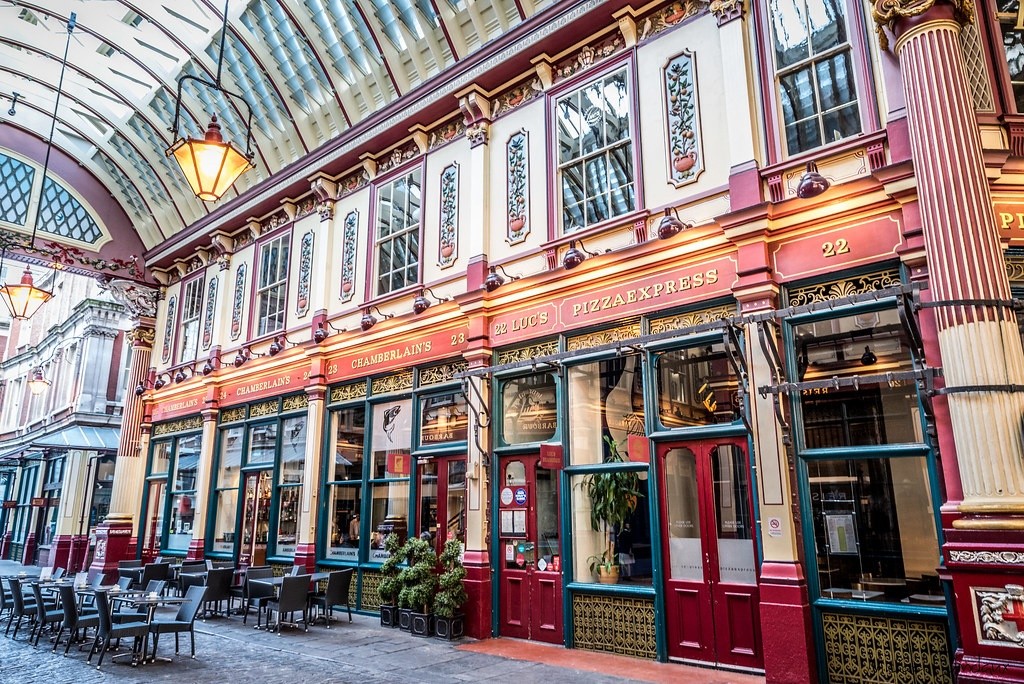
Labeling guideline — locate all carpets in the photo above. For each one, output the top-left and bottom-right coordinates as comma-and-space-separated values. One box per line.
450, 637, 766, 684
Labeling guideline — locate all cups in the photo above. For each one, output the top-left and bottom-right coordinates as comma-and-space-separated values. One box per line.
218, 567, 224, 569
44, 576, 50, 579
78, 582, 86, 586
285, 573, 290, 577
19, 572, 25, 574
55, 579, 62, 582
113, 584, 120, 589
149, 592, 158, 596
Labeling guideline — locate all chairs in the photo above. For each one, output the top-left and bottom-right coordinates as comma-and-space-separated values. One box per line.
817, 557, 946, 605
0, 557, 353, 670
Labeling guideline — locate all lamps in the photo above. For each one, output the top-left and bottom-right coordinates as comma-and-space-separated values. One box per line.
861, 498, 870, 508
165, 0, 258, 202
28, 337, 84, 395
135, 159, 833, 396
96, 483, 103, 490
704, 390, 717, 407
695, 380, 709, 394
860, 346, 878, 366
0, 12, 87, 322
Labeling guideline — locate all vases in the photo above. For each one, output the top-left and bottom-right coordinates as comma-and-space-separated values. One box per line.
222, 533, 234, 543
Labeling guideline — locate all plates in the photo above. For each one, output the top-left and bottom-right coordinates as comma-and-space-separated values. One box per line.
78, 586, 88, 588
147, 596, 160, 599
110, 589, 122, 592
55, 582, 64, 584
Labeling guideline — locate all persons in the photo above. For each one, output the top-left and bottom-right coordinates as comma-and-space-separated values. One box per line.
371, 532, 382, 550
333, 513, 361, 547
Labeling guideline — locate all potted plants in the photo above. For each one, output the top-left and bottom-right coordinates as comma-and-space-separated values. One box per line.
377, 531, 468, 642
572, 436, 644, 583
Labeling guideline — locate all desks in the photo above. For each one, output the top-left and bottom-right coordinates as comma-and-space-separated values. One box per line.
77, 589, 151, 652
859, 577, 922, 601
164, 563, 184, 605
26, 582, 76, 636
296, 572, 339, 626
248, 576, 299, 632
222, 568, 248, 616
19, 579, 70, 632
179, 571, 225, 622
110, 596, 193, 665
118, 567, 157, 609
49, 584, 121, 645
1, 575, 40, 623
824, 587, 886, 602
